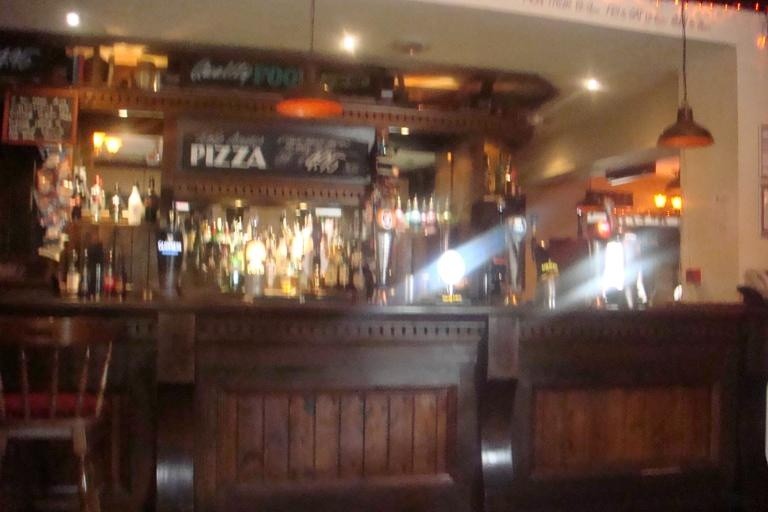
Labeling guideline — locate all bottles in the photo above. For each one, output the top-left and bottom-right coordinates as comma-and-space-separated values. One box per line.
59, 173, 456, 305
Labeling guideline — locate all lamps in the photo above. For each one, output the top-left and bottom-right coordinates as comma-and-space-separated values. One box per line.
276, 0, 344, 119
657, 6, 715, 150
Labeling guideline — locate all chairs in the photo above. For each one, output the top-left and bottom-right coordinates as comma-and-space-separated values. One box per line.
1, 314, 123, 511
